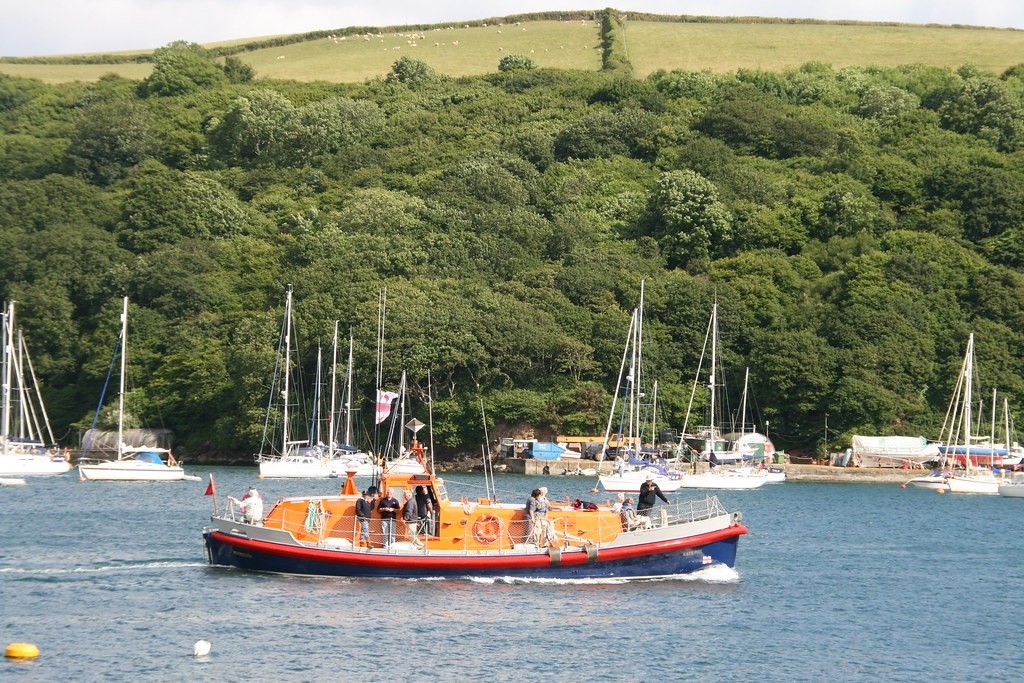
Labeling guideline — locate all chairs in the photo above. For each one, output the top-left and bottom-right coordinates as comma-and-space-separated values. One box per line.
621, 514, 637, 532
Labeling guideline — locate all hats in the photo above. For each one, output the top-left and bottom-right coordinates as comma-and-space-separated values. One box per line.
645, 475, 654, 480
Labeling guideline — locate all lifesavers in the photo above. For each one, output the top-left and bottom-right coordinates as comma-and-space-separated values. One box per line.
346, 461, 360, 470
764, 442, 772, 452
472, 512, 503, 544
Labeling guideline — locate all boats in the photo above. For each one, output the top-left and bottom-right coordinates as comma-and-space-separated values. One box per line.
203, 420, 750, 589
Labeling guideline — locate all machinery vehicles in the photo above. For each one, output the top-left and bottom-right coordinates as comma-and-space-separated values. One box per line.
557, 432, 741, 469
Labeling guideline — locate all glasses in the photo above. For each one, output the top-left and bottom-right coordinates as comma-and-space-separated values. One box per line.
363, 494, 368, 495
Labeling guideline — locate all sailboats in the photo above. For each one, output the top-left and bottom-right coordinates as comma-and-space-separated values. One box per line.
0, 298, 87, 484
78, 297, 188, 481
255, 283, 421, 478
598, 282, 793, 494
908, 331, 1024, 500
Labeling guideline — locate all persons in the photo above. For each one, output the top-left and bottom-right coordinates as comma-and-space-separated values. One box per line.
415, 485, 435, 535
524, 487, 563, 548
228, 487, 263, 525
377, 487, 400, 549
637, 474, 672, 516
356, 490, 377, 548
401, 490, 425, 550
620, 497, 651, 532
612, 493, 625, 513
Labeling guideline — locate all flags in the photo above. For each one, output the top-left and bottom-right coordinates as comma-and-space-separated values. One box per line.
376, 389, 398, 424
205, 479, 215, 495
